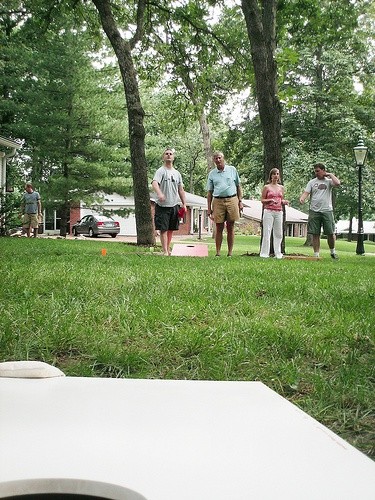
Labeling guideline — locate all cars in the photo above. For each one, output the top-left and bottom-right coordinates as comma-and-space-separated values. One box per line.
71, 214, 121, 239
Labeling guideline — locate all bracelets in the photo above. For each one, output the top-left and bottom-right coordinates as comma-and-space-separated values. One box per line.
38, 212, 42, 213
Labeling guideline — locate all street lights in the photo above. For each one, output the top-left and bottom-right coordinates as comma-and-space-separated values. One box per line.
352, 138, 369, 255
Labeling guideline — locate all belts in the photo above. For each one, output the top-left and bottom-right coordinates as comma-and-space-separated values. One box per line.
215, 195, 236, 198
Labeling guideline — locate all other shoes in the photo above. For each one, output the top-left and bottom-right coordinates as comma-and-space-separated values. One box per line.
330, 253, 338, 259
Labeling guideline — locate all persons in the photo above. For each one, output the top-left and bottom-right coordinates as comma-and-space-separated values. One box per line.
260, 168, 289, 259
151, 149, 187, 256
19, 183, 42, 238
299, 163, 341, 259
206, 151, 244, 257
